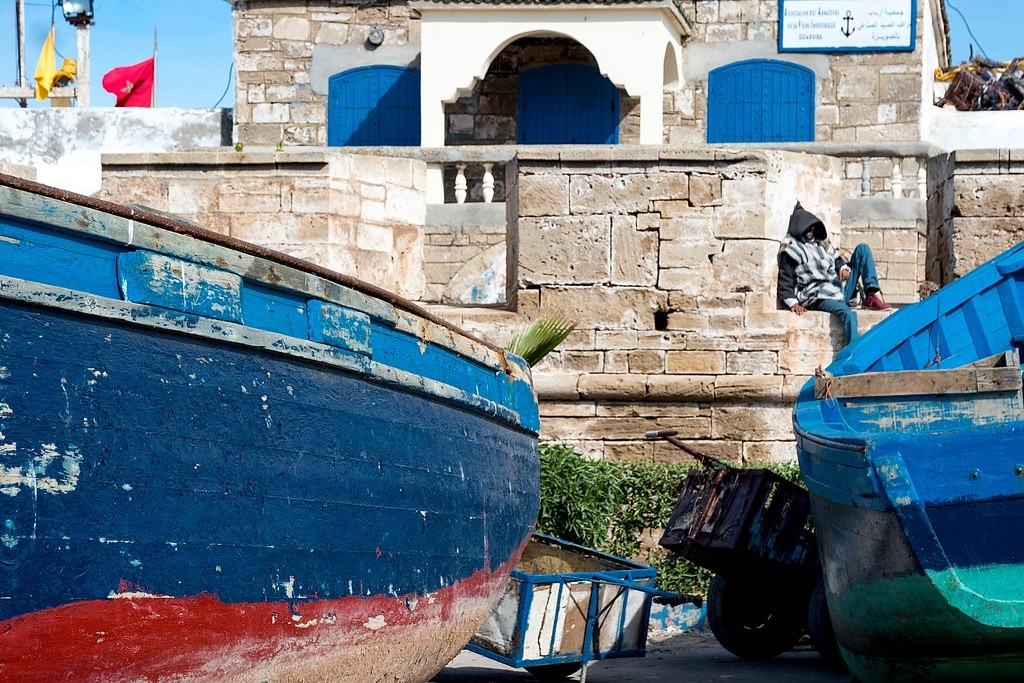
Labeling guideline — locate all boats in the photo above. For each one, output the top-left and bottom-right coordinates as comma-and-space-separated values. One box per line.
793, 242, 1023, 683
0, 173, 542, 683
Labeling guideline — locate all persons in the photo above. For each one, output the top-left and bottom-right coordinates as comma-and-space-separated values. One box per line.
778, 204, 890, 350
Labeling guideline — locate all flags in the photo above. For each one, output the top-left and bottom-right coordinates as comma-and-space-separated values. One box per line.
103, 58, 154, 108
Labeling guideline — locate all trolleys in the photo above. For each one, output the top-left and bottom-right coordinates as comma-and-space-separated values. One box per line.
645, 426, 849, 672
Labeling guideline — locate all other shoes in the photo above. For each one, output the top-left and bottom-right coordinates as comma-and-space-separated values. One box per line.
861, 292, 893, 311
